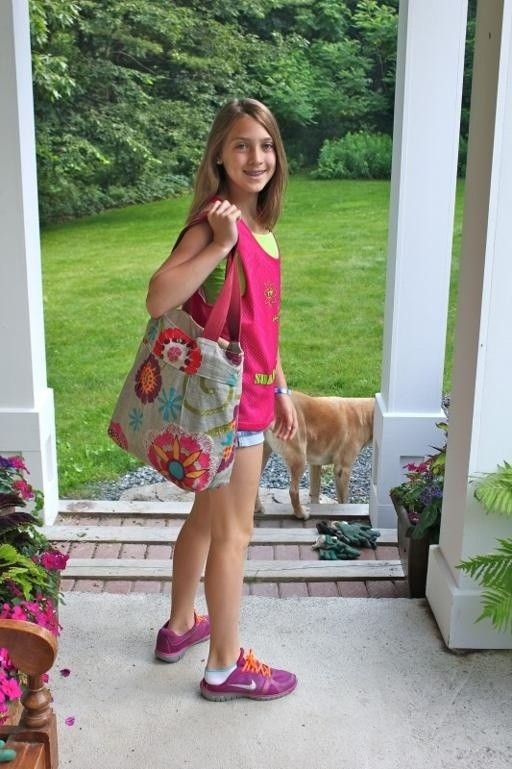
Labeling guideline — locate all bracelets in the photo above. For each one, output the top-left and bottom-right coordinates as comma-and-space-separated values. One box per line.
275, 387, 293, 396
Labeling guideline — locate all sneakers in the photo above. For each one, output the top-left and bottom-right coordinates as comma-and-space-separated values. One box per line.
201, 647, 297, 701
154, 609, 210, 662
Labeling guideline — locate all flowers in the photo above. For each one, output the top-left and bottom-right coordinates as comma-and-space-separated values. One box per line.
0, 456, 68, 725
390, 397, 448, 541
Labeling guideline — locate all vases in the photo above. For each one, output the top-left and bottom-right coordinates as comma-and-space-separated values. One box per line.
389, 489, 440, 597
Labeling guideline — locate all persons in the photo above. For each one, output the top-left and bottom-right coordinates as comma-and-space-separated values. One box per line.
144, 93, 297, 701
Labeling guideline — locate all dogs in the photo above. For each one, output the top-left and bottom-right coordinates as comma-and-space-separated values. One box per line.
257, 388, 376, 522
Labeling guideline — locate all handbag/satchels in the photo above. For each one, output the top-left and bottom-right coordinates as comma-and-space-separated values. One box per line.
108, 212, 244, 493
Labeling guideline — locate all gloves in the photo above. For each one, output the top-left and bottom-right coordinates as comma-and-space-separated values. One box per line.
312, 519, 381, 560
